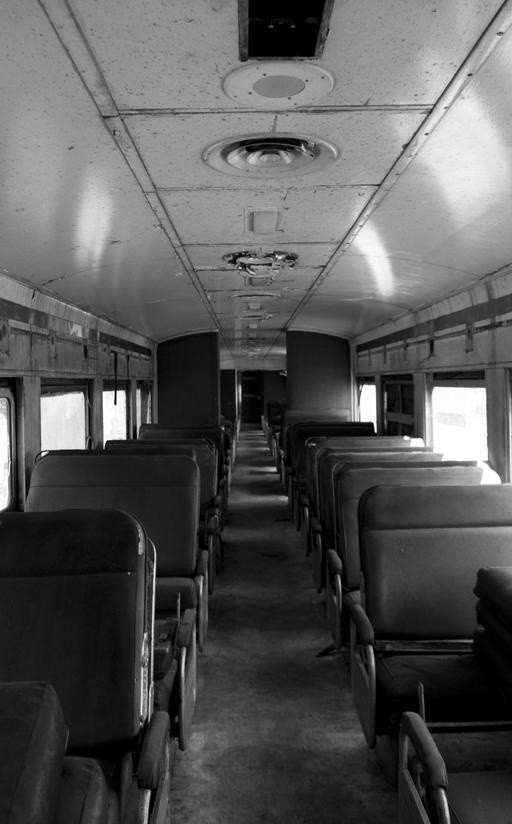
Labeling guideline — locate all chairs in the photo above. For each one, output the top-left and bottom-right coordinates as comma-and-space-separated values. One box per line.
0, 414, 236, 824
261, 400, 512, 824
